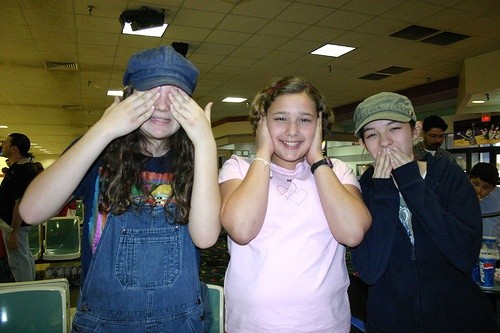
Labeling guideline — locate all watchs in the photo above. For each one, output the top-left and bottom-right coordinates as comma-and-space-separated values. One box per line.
310, 156, 334, 174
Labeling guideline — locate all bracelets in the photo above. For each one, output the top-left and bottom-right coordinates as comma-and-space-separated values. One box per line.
249, 157, 272, 166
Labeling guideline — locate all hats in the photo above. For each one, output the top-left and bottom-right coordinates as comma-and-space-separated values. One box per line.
353, 91, 416, 138
122, 46, 200, 98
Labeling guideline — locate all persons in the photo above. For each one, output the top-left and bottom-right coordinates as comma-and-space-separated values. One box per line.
0, 132, 42, 283
414, 116, 459, 164
18, 45, 221, 333
216, 75, 375, 333
467, 162, 500, 237
347, 92, 500, 333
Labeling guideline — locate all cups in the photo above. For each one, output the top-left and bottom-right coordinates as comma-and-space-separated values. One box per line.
479, 255, 496, 289
483, 236, 496, 255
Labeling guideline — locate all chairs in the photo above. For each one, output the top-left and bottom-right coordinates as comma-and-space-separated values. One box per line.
43, 216, 82, 262
76, 200, 85, 224
27, 224, 42, 260
0, 278, 70, 333
202, 284, 225, 333
